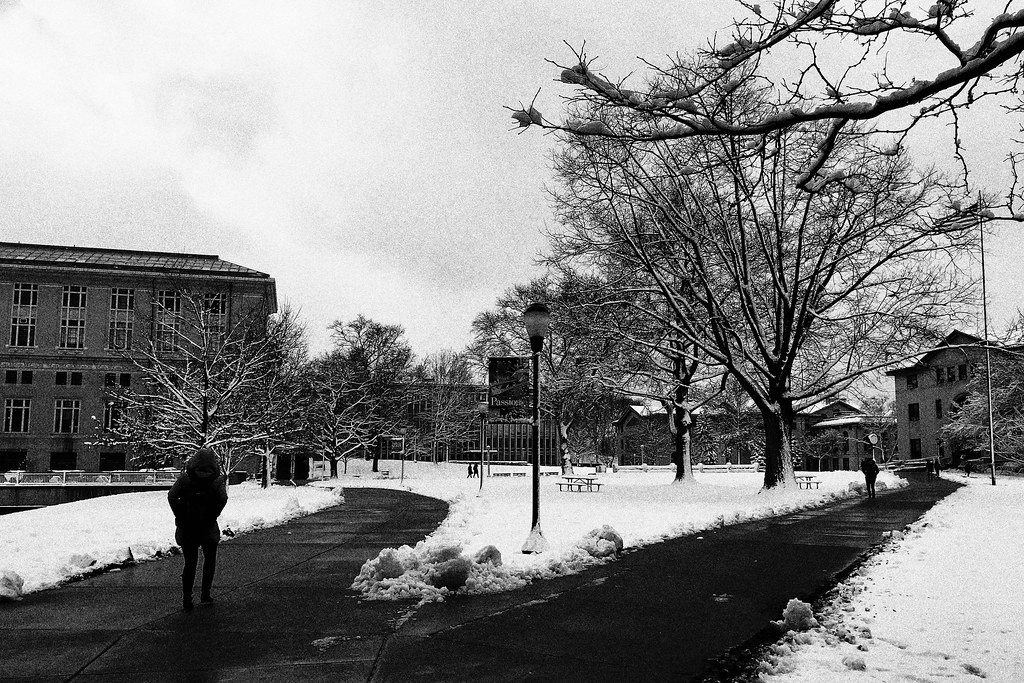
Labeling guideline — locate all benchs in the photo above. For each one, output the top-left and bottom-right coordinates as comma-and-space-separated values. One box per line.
797, 481, 822, 484
576, 481, 604, 486
555, 482, 586, 487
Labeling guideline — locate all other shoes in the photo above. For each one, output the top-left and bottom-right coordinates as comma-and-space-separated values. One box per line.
183, 602, 194, 610
200, 594, 214, 603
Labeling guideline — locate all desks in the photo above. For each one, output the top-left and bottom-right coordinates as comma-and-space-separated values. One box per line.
559, 476, 600, 492
795, 476, 820, 489
9, 470, 27, 485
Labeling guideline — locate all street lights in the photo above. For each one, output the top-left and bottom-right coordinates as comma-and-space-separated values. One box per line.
477, 399, 490, 489
521, 302, 552, 555
399, 423, 408, 484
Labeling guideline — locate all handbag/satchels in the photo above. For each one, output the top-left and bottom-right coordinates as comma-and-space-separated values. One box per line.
175, 526, 181, 546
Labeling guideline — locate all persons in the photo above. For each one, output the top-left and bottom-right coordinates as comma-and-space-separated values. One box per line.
168, 449, 228, 612
467, 462, 479, 478
965, 462, 971, 477
926, 458, 940, 481
861, 454, 879, 499
